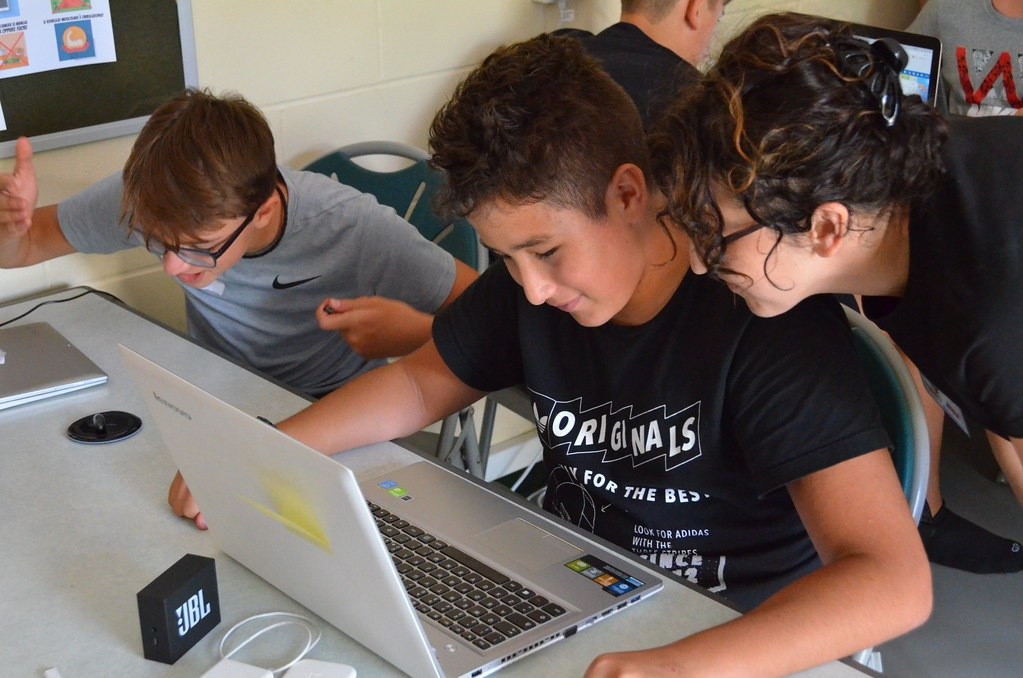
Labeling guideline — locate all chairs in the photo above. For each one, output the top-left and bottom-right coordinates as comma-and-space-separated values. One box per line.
831, 295, 932, 671
295, 140, 489, 487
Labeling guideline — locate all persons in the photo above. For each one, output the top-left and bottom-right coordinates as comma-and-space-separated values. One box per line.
167, 0, 1023, 678
0, 87, 481, 401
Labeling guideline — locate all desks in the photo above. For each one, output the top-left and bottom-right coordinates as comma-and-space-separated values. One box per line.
0, 282, 894, 678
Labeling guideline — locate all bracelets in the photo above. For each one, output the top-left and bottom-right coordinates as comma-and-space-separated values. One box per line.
256, 415, 279, 432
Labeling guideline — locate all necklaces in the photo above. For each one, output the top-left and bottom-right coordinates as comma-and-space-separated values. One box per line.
241, 182, 289, 259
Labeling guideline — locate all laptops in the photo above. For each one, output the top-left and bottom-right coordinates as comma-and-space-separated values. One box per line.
0, 322, 109, 410
786, 11, 942, 108
118, 343, 663, 677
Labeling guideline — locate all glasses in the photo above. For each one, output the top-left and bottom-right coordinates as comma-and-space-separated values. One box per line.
128, 205, 260, 269
669, 200, 804, 267
